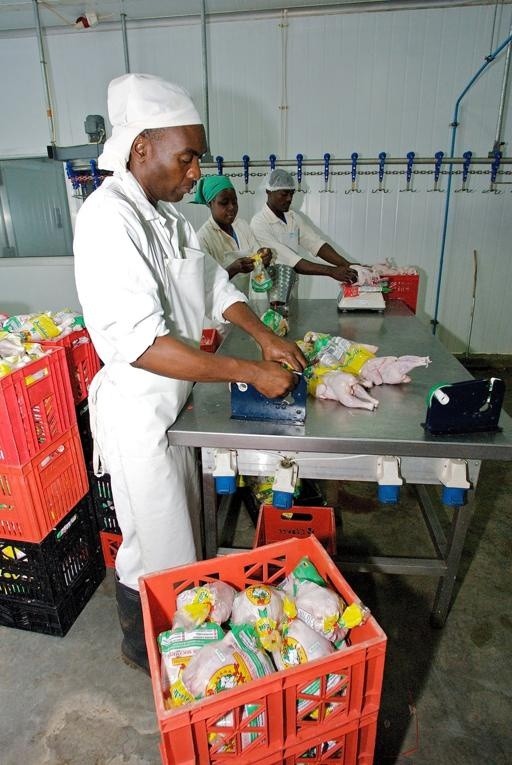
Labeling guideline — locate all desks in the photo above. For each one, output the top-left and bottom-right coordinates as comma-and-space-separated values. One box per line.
163, 298, 512, 632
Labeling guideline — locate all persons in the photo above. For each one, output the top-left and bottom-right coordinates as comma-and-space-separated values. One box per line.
191, 172, 276, 352
71, 73, 313, 682
248, 168, 371, 303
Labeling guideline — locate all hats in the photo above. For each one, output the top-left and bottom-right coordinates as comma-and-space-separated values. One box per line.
105, 72, 203, 128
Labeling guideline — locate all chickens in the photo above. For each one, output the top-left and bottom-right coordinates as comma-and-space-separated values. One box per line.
313, 369, 383, 412
359, 351, 433, 388
346, 253, 418, 289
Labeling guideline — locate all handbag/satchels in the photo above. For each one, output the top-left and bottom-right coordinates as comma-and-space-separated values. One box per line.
422, 376, 505, 435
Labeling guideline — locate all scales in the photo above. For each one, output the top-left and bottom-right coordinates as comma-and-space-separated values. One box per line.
336, 291, 386, 313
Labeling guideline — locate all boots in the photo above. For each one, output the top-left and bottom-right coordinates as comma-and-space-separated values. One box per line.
113, 568, 151, 676
